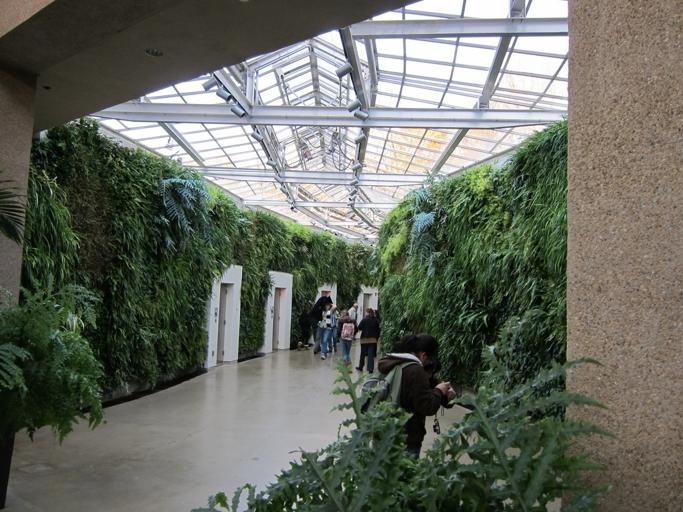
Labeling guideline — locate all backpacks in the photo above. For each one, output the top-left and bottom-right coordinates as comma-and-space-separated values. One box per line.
360, 361, 417, 421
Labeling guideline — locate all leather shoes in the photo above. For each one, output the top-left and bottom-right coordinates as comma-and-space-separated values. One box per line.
356, 366, 362, 370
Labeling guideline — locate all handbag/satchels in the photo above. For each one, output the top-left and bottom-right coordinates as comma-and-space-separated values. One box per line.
341, 322, 355, 341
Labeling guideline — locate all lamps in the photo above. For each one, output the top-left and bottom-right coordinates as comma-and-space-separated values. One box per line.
201, 63, 368, 220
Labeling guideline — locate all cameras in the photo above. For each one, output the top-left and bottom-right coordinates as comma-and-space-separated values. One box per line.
431, 421, 441, 434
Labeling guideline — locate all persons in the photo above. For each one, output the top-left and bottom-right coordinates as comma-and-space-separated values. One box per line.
336, 310, 357, 368
346, 303, 359, 341
372, 309, 382, 358
421, 356, 457, 403
375, 331, 451, 482
297, 289, 339, 360
356, 308, 380, 373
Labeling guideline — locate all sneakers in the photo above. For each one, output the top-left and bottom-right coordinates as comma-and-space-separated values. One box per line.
343, 359, 351, 366
321, 347, 337, 360
